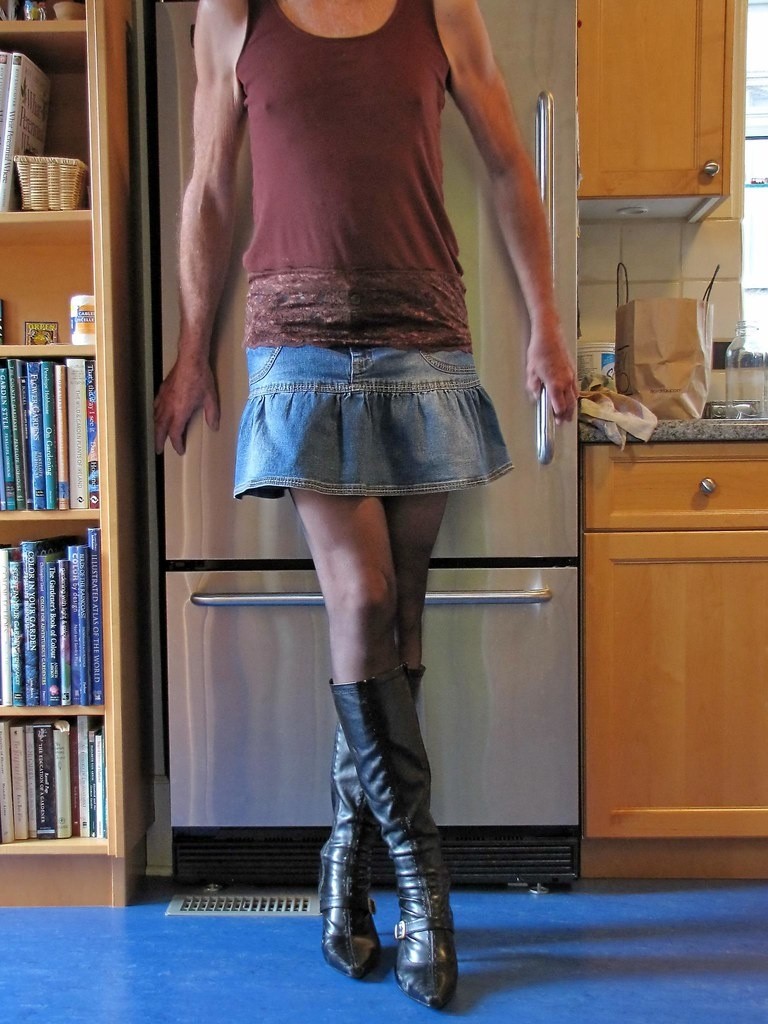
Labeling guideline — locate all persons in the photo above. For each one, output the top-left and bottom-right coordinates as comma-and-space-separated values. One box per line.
153, 0, 579, 1008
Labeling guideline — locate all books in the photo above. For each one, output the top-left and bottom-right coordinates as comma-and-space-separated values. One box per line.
0, 356, 112, 843
0, 52, 52, 210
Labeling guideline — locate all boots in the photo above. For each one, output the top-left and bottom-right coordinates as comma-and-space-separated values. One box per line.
328, 661, 458, 1010
317, 663, 428, 978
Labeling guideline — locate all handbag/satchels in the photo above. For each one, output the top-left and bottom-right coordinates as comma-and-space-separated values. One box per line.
608, 259, 720, 418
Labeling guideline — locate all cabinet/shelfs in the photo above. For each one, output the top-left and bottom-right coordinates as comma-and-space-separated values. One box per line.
576, 442, 767, 877
0, 0, 167, 907
576, 0, 736, 225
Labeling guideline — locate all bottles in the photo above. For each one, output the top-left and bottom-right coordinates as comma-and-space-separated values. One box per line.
725, 322, 768, 418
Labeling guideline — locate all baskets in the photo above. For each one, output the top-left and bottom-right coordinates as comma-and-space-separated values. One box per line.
12, 154, 89, 211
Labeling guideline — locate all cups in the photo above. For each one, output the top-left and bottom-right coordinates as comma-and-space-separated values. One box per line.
580, 342, 614, 380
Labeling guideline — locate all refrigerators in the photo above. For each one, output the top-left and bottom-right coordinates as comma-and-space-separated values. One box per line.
156, 0, 579, 894
